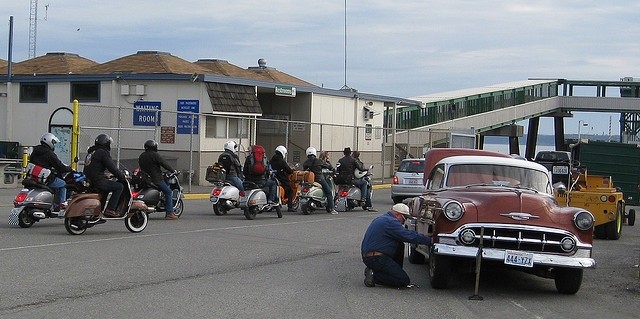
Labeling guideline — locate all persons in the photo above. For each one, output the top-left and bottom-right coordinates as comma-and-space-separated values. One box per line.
336, 148, 369, 209
84, 133, 125, 217
269, 145, 296, 212
319, 150, 337, 173
21, 131, 71, 211
303, 146, 339, 214
139, 140, 179, 220
218, 140, 244, 192
351, 150, 366, 169
243, 146, 277, 206
361, 203, 431, 290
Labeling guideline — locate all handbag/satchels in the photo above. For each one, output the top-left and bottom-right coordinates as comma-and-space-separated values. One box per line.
25, 156, 53, 183
293, 158, 316, 184
206, 162, 226, 181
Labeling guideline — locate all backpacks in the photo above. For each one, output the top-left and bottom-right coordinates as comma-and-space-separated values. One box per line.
248, 144, 267, 175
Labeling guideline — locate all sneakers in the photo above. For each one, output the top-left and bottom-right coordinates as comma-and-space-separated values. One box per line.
105, 208, 121, 217
364, 268, 375, 287
165, 214, 177, 220
267, 200, 279, 206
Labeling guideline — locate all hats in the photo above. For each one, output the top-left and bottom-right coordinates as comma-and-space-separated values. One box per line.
390, 203, 410, 216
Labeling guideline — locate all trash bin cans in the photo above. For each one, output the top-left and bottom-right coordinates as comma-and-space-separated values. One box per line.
182, 169, 195, 185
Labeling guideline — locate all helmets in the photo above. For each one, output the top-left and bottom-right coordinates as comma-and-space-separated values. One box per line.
95, 134, 113, 146
275, 146, 287, 159
306, 147, 317, 159
144, 140, 159, 151
40, 133, 61, 150
224, 140, 238, 153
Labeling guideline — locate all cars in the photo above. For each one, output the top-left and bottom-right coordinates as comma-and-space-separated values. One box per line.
402, 147, 595, 294
391, 159, 431, 204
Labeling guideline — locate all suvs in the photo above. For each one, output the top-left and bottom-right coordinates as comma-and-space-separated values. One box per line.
531, 151, 570, 185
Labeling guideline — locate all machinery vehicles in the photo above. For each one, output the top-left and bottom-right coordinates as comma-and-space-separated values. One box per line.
552, 159, 635, 239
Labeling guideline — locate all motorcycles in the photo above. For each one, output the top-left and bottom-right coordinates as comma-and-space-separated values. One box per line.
210, 180, 245, 216
239, 161, 283, 220
334, 165, 375, 211
298, 163, 341, 215
130, 170, 184, 218
62, 169, 149, 235
8, 157, 86, 228
280, 163, 300, 212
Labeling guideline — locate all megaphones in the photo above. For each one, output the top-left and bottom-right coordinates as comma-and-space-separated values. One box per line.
367, 100, 374, 108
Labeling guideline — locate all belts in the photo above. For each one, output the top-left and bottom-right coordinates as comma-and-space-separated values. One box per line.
364, 251, 384, 257
